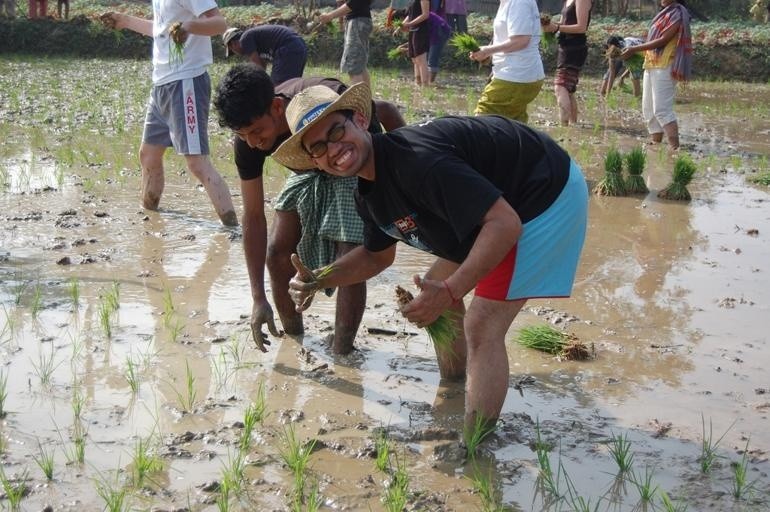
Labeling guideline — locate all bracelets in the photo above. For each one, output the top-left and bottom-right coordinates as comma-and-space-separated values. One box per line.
442, 277, 459, 305
553, 25, 559, 33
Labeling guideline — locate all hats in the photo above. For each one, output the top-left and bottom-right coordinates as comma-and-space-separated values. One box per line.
270, 81, 372, 170
221, 27, 240, 58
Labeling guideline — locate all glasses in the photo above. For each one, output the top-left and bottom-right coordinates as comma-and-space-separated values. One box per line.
304, 112, 353, 158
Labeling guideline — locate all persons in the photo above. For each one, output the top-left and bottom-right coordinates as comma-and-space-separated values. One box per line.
27, 2, 50, 22
223, 24, 311, 88
385, 1, 468, 86
1, 0, 18, 21
319, 1, 372, 84
57, 1, 71, 21
213, 66, 411, 362
543, 1, 593, 125
268, 80, 590, 448
601, 36, 647, 96
469, 1, 547, 122
618, 2, 689, 150
85, 1, 241, 233
